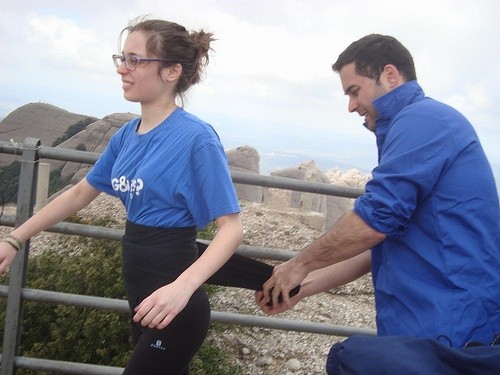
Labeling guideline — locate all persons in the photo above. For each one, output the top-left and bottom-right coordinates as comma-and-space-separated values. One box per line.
0, 14, 244, 375
255, 32, 500, 375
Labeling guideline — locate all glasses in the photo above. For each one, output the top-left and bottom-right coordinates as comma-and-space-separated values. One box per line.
113, 55, 176, 71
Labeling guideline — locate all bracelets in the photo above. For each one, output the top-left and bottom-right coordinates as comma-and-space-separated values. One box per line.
3, 235, 23, 251
